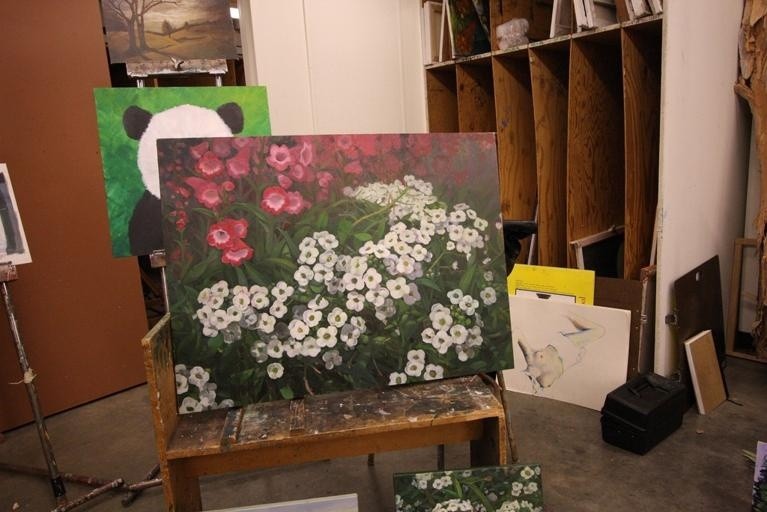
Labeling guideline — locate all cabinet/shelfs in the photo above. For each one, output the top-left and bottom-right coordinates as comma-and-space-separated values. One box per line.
420, 0, 752, 379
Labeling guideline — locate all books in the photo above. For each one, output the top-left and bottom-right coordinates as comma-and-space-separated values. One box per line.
684, 329, 727, 415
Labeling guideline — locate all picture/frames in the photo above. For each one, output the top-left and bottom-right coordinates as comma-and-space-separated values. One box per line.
549, 0, 663, 38
568, 226, 625, 278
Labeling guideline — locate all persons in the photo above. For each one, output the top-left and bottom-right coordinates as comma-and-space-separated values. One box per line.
513, 310, 607, 391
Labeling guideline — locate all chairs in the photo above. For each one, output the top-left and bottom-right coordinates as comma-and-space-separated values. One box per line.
140, 249, 508, 511
